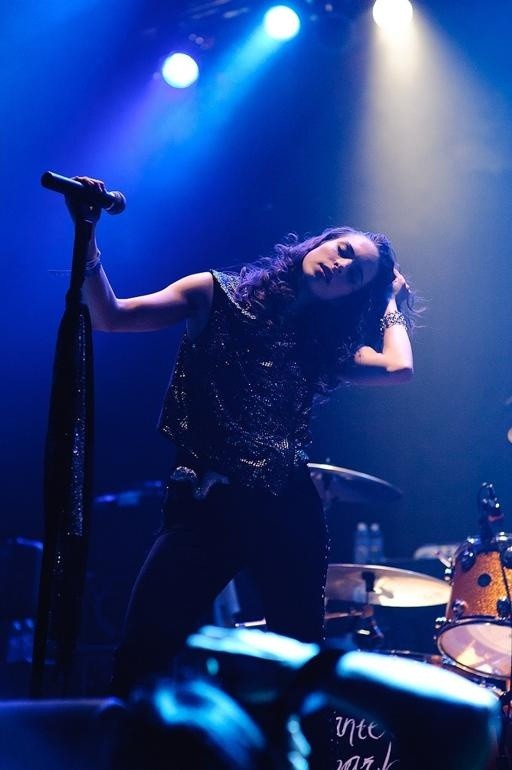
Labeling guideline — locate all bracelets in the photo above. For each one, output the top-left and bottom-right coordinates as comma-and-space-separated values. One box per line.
379, 310, 407, 330
81, 247, 104, 276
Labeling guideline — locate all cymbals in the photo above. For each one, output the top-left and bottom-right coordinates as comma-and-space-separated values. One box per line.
307, 462, 402, 513
326, 562, 453, 608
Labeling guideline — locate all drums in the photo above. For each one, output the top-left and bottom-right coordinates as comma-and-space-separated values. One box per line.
433, 531, 512, 681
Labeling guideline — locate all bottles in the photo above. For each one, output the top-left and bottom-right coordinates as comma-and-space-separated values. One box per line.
6, 618, 35, 664
353, 522, 370, 564
370, 523, 386, 564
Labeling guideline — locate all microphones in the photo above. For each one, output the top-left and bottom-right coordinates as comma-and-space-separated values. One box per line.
41, 171, 126, 215
488, 486, 504, 536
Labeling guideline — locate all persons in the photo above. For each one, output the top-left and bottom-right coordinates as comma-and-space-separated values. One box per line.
65, 174, 417, 697
